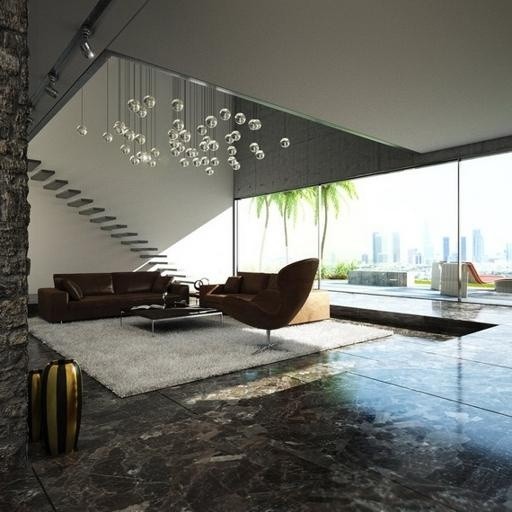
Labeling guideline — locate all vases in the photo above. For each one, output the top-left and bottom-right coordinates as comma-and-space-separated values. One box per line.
24, 368, 46, 444
38, 357, 84, 454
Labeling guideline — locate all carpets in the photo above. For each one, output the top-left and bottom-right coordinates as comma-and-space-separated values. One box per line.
26, 299, 398, 398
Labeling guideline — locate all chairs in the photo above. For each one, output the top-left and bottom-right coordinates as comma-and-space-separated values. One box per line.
218, 256, 319, 354
459, 257, 505, 294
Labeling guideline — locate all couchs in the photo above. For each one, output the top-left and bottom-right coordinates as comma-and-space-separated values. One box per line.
37, 271, 181, 325
196, 271, 277, 309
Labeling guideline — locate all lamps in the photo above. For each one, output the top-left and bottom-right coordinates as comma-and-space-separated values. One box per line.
45, 70, 62, 98
73, 28, 96, 63
76, 55, 294, 178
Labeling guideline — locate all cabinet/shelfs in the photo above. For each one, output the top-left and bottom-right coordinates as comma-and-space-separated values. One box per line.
344, 270, 409, 288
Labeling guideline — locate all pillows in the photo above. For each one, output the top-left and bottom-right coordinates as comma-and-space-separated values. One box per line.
151, 273, 173, 292
52, 276, 84, 300
220, 275, 244, 295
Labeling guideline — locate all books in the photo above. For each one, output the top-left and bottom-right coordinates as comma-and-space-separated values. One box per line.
131, 303, 166, 311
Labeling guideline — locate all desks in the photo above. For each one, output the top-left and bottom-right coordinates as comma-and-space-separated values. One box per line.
287, 286, 331, 325
494, 277, 512, 297
